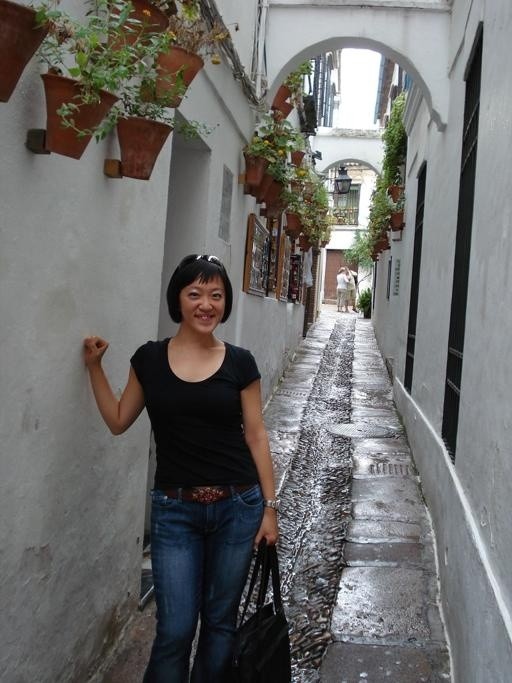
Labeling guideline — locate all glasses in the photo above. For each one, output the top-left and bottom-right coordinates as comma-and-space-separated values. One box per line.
184, 254, 220, 264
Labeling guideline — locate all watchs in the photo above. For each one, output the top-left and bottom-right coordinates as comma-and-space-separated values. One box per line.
262, 499, 282, 511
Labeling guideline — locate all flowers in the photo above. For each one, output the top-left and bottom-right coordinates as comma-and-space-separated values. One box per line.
242, 121, 338, 251
0, 0, 239, 140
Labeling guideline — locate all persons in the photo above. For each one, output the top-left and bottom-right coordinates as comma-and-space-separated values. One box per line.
336, 265, 358, 313
84, 254, 289, 683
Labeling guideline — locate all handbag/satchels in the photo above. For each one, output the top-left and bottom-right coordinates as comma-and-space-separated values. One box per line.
226, 537, 291, 683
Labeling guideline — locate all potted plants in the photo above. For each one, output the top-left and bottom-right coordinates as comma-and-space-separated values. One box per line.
342, 89, 409, 273
272, 59, 315, 121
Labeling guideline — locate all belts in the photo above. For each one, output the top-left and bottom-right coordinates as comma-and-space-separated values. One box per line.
162, 481, 249, 503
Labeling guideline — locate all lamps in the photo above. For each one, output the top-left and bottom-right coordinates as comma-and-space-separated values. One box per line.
334, 165, 353, 194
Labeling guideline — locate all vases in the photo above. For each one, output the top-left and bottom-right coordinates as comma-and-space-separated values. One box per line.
41, 73, 118, 162
118, 114, 175, 181
